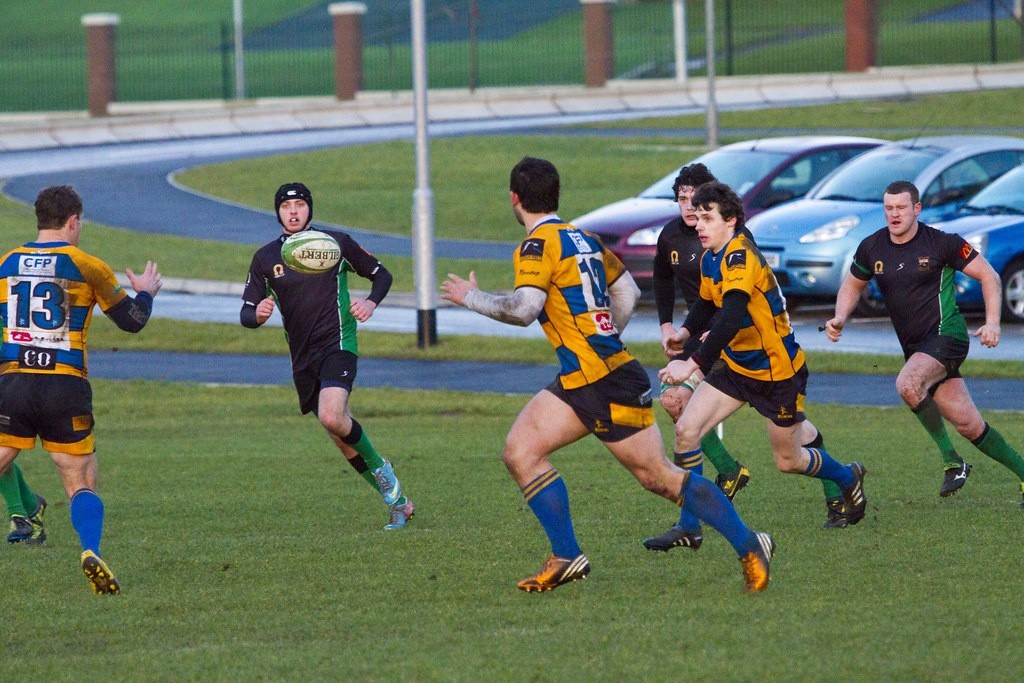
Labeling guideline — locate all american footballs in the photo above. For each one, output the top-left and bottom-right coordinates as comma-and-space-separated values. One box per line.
281, 230, 342, 272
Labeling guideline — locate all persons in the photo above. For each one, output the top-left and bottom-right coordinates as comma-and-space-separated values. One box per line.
439, 156, 776, 591
0, 183, 161, 594
643, 183, 866, 553
826, 181, 1023, 506
654, 162, 847, 529
241, 182, 417, 531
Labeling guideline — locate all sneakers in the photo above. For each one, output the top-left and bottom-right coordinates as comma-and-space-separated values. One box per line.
716, 462, 750, 501
515, 552, 592, 592
842, 461, 867, 525
7, 494, 48, 544
823, 496, 848, 528
739, 533, 777, 594
383, 497, 414, 531
643, 527, 705, 551
369, 458, 402, 505
938, 458, 972, 497
1017, 482, 1024, 509
80, 549, 121, 594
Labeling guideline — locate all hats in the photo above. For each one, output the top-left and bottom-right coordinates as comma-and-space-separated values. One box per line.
273, 182, 313, 233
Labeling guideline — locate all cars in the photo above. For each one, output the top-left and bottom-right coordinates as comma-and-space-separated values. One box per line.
744, 135, 1024, 317
569, 134, 895, 302
864, 162, 1024, 323
